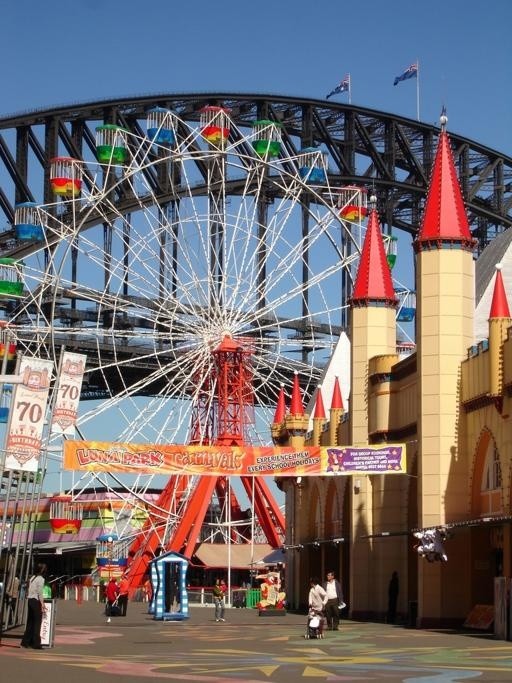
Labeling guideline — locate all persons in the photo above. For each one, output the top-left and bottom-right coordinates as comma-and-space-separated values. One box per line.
20, 562, 52, 650
105, 573, 130, 616
6, 574, 21, 625
307, 569, 344, 634
213, 577, 228, 622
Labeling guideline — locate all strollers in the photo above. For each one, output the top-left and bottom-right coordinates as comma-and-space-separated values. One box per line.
303, 611, 328, 641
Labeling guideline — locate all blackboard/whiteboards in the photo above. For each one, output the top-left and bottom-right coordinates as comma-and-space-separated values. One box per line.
39, 599, 55, 645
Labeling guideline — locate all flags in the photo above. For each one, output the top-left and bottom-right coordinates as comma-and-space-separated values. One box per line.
325, 73, 350, 101
393, 64, 417, 85
